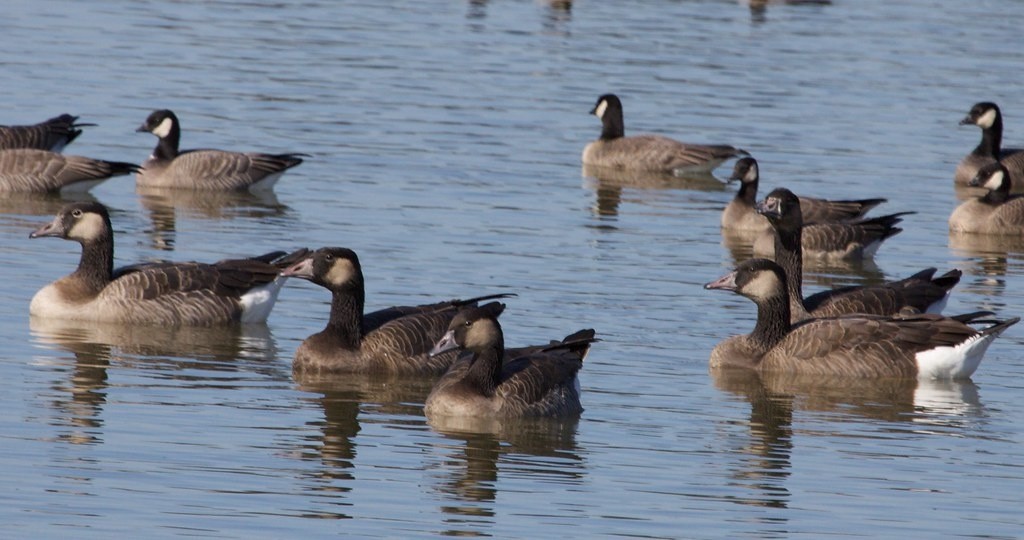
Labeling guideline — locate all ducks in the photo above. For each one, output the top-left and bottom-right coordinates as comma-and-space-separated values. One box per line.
0, 94, 1024, 418
